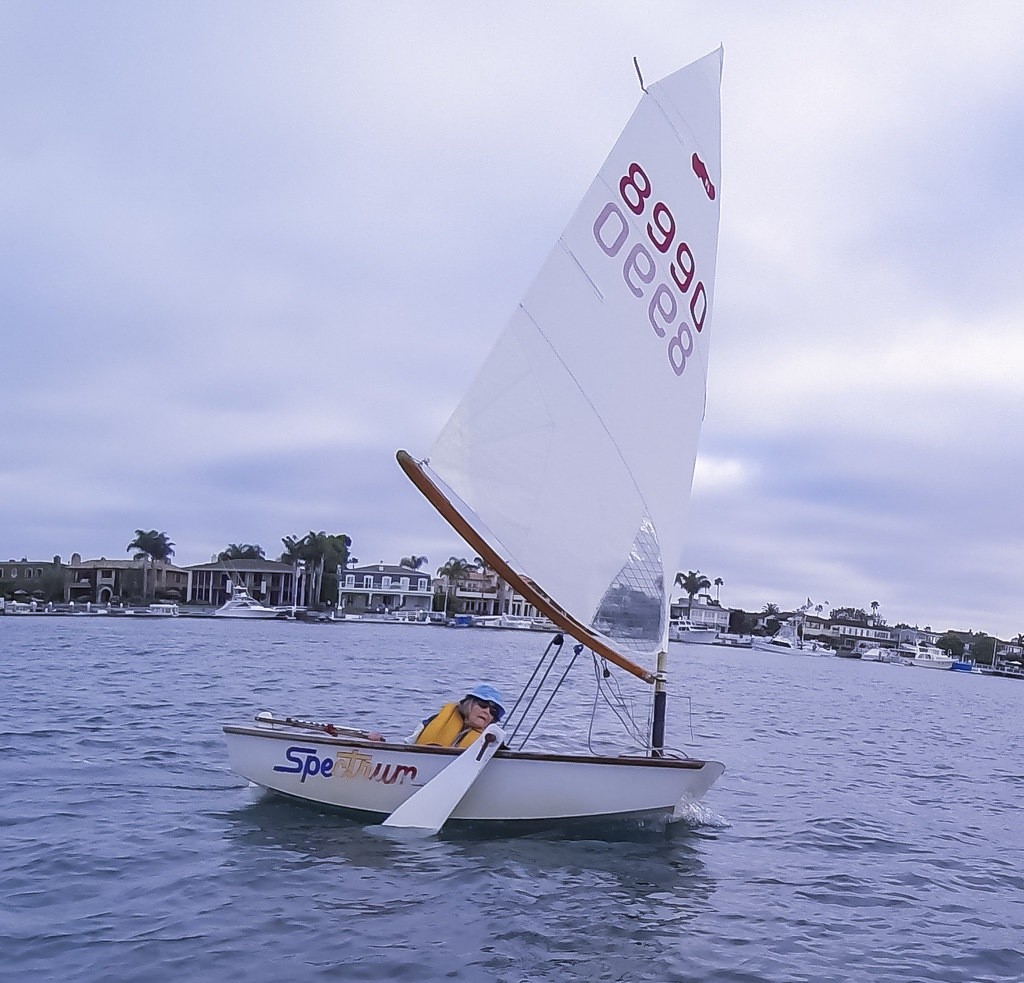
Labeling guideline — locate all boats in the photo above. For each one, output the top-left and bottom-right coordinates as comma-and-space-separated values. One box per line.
667, 617, 721, 644
723, 610, 984, 675
445, 611, 567, 636
213, 585, 288, 619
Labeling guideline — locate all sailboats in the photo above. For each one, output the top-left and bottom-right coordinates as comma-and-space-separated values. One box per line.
219, 30, 731, 836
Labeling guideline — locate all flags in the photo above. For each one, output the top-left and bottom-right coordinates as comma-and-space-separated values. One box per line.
297, 559, 306, 570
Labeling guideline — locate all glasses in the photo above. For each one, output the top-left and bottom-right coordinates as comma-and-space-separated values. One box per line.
477, 699, 499, 717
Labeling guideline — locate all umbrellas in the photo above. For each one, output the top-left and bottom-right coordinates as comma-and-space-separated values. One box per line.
166, 590, 179, 600
14, 589, 28, 595
32, 589, 45, 594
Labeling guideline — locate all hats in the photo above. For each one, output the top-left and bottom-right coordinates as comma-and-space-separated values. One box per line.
467, 682, 505, 718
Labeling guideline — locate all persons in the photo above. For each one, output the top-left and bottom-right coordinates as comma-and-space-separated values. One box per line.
327, 599, 331, 609
366, 684, 505, 748
813, 644, 817, 651
801, 642, 803, 649
